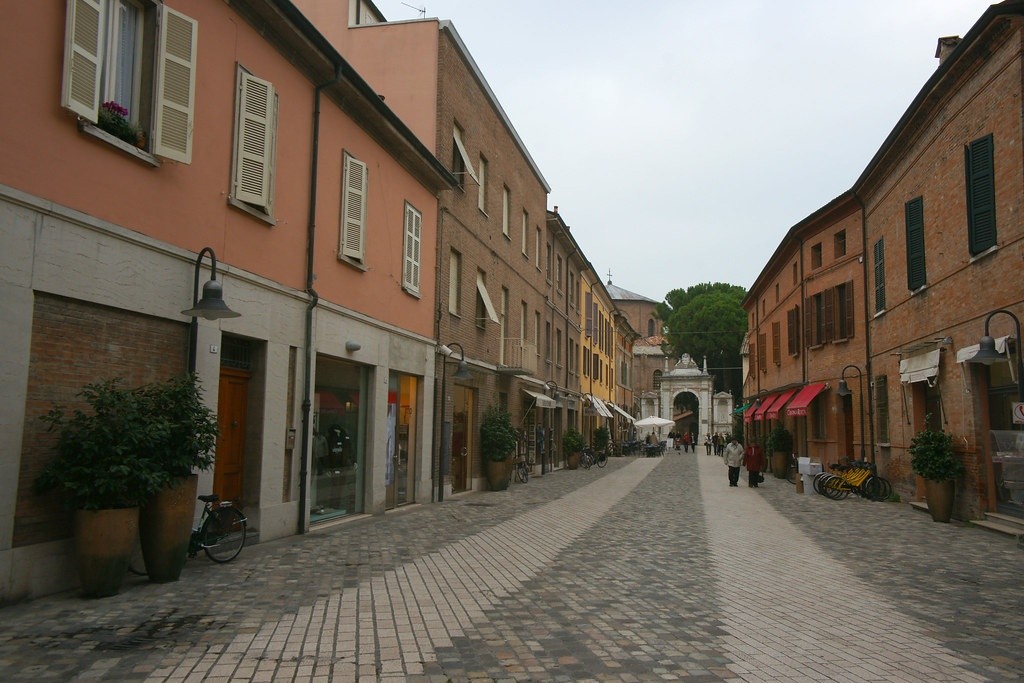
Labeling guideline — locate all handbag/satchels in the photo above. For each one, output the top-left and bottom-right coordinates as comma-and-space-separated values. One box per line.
758, 472, 764, 483
704, 442, 707, 445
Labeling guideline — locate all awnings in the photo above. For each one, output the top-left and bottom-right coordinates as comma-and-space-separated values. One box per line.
522, 388, 556, 409
786, 383, 825, 415
610, 402, 636, 420
453, 135, 481, 186
729, 386, 798, 421
476, 270, 501, 325
586, 395, 613, 418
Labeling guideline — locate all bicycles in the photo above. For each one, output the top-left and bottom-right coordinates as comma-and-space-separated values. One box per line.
512, 452, 531, 483
191, 493, 248, 563
579, 444, 608, 471
813, 455, 892, 502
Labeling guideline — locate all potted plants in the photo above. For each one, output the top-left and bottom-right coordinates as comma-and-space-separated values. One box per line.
561, 427, 585, 470
766, 425, 792, 479
907, 412, 965, 522
36, 365, 225, 599
478, 405, 523, 491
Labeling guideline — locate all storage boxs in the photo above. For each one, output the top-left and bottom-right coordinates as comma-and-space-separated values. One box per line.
797, 456, 822, 475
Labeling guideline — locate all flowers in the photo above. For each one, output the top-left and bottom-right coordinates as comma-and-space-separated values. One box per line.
97, 100, 144, 134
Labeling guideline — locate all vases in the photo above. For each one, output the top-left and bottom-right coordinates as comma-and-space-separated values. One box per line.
89, 113, 137, 146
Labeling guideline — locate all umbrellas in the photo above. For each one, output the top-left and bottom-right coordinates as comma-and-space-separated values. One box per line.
634, 416, 676, 434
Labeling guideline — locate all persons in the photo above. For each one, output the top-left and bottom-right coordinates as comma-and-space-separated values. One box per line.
650, 432, 657, 445
742, 439, 766, 489
311, 424, 351, 514
667, 429, 697, 455
722, 435, 744, 487
704, 432, 732, 457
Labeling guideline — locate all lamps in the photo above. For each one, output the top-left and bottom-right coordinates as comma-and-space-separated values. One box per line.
941, 335, 953, 345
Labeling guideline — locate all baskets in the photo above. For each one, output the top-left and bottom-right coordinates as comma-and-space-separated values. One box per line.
514, 456, 526, 464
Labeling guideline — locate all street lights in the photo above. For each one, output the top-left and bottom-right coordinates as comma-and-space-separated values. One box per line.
834, 365, 865, 468
542, 380, 563, 475
438, 343, 475, 502
756, 389, 772, 473
968, 310, 1023, 403
581, 392, 595, 446
181, 246, 243, 386
